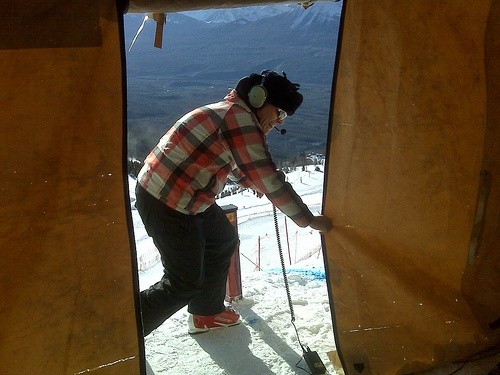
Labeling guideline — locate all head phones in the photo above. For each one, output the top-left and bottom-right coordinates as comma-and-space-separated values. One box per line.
248, 72, 269, 108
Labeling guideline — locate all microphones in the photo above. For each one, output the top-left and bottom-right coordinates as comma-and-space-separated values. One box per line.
274, 126, 287, 135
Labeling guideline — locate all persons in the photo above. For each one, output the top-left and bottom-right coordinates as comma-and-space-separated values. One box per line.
134, 70, 333, 337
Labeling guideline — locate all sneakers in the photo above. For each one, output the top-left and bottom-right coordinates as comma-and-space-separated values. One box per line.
191, 307, 240, 328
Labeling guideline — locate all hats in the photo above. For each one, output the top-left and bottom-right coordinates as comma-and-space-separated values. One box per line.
250, 69, 303, 115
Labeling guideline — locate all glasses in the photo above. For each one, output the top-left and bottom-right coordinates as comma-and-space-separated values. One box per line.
272, 103, 286, 121
